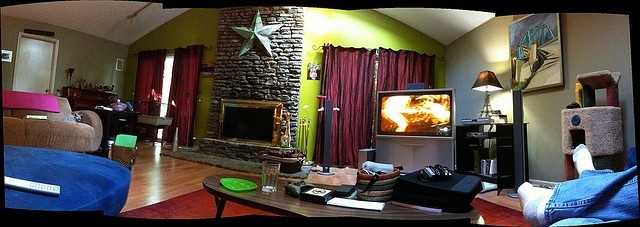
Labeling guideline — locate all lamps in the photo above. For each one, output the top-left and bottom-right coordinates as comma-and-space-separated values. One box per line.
69, 68, 74, 87
471, 71, 503, 118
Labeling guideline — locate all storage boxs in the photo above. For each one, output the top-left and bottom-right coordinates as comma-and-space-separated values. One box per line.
266, 155, 304, 173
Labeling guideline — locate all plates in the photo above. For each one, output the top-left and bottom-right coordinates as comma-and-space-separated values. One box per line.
220, 177, 257, 191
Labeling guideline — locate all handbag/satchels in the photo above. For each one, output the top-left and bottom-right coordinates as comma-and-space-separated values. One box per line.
392, 167, 483, 213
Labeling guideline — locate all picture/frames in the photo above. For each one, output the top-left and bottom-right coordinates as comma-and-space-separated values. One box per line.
508, 13, 564, 92
1, 50, 13, 63
116, 58, 124, 71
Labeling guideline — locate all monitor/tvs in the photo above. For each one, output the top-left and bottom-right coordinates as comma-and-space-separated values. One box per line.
374, 88, 456, 139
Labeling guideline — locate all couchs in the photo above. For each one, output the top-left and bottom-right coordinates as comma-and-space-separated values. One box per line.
4, 146, 130, 217
2, 89, 103, 153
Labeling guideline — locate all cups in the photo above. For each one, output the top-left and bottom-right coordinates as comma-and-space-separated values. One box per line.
262, 161, 281, 193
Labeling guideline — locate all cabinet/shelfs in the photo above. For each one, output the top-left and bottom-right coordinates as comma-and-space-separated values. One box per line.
64, 87, 119, 112
455, 123, 529, 197
376, 136, 454, 173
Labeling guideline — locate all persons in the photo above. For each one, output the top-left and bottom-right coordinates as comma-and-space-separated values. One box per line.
517, 143, 640, 225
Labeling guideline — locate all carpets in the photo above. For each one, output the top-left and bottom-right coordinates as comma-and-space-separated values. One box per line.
471, 198, 531, 226
119, 189, 534, 227
162, 150, 312, 178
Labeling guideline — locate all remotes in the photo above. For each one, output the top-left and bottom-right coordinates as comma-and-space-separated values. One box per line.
4, 175, 62, 198
336, 184, 354, 196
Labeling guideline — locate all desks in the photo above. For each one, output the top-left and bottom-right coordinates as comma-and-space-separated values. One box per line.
139, 115, 173, 147
81, 107, 139, 151
561, 105, 622, 182
202, 174, 482, 219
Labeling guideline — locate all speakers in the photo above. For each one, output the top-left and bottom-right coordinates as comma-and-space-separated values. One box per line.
507, 90, 526, 198
317, 99, 336, 175
